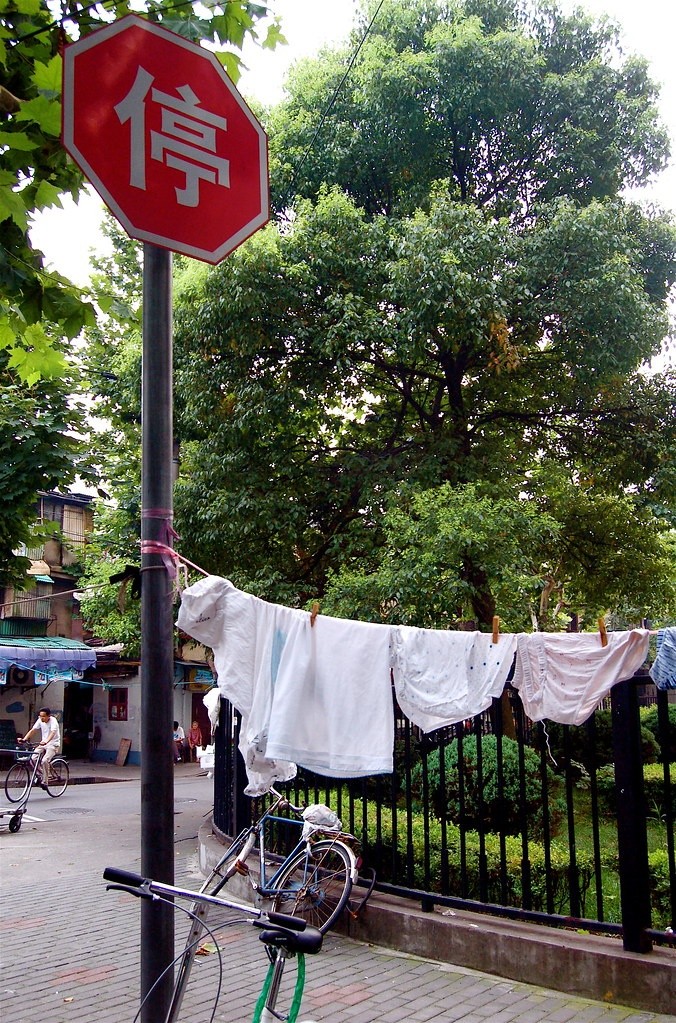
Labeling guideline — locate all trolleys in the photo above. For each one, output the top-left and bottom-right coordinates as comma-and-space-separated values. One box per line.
0, 749, 42, 833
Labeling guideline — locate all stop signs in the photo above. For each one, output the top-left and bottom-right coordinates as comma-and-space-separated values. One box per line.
61, 12, 272, 265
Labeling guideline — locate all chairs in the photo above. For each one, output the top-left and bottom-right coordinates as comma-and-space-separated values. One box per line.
0, 719, 21, 754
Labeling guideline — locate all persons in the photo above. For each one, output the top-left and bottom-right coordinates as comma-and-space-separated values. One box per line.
17, 707, 61, 790
173, 721, 185, 767
188, 721, 202, 763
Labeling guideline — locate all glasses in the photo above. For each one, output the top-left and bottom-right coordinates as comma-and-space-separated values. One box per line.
38, 716, 45, 719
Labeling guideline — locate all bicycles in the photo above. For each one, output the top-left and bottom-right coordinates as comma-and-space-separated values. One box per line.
2, 740, 71, 803
187, 781, 361, 945
99, 865, 325, 1021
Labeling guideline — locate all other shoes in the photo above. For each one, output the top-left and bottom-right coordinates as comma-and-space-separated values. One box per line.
196, 759, 200, 763
176, 757, 182, 761
41, 782, 48, 786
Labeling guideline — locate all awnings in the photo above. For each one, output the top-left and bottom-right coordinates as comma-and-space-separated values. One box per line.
175, 660, 209, 666
0, 635, 97, 674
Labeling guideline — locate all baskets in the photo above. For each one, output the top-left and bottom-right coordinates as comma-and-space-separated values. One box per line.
15, 745, 35, 765
